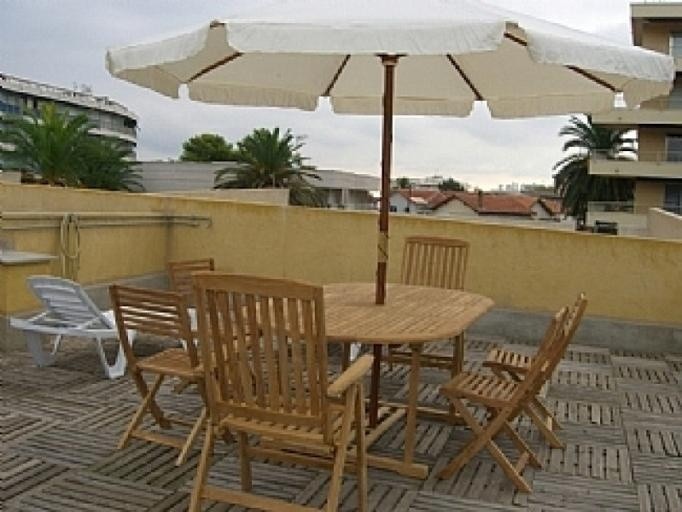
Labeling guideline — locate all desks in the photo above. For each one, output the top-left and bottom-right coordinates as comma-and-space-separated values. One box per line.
241, 283, 495, 479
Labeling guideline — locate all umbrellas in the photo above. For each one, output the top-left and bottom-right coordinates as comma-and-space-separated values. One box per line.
104, 0, 677, 427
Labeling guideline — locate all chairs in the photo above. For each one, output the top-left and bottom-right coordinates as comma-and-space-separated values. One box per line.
108, 272, 376, 511
436, 292, 587, 493
9, 257, 215, 380
388, 236, 470, 379
